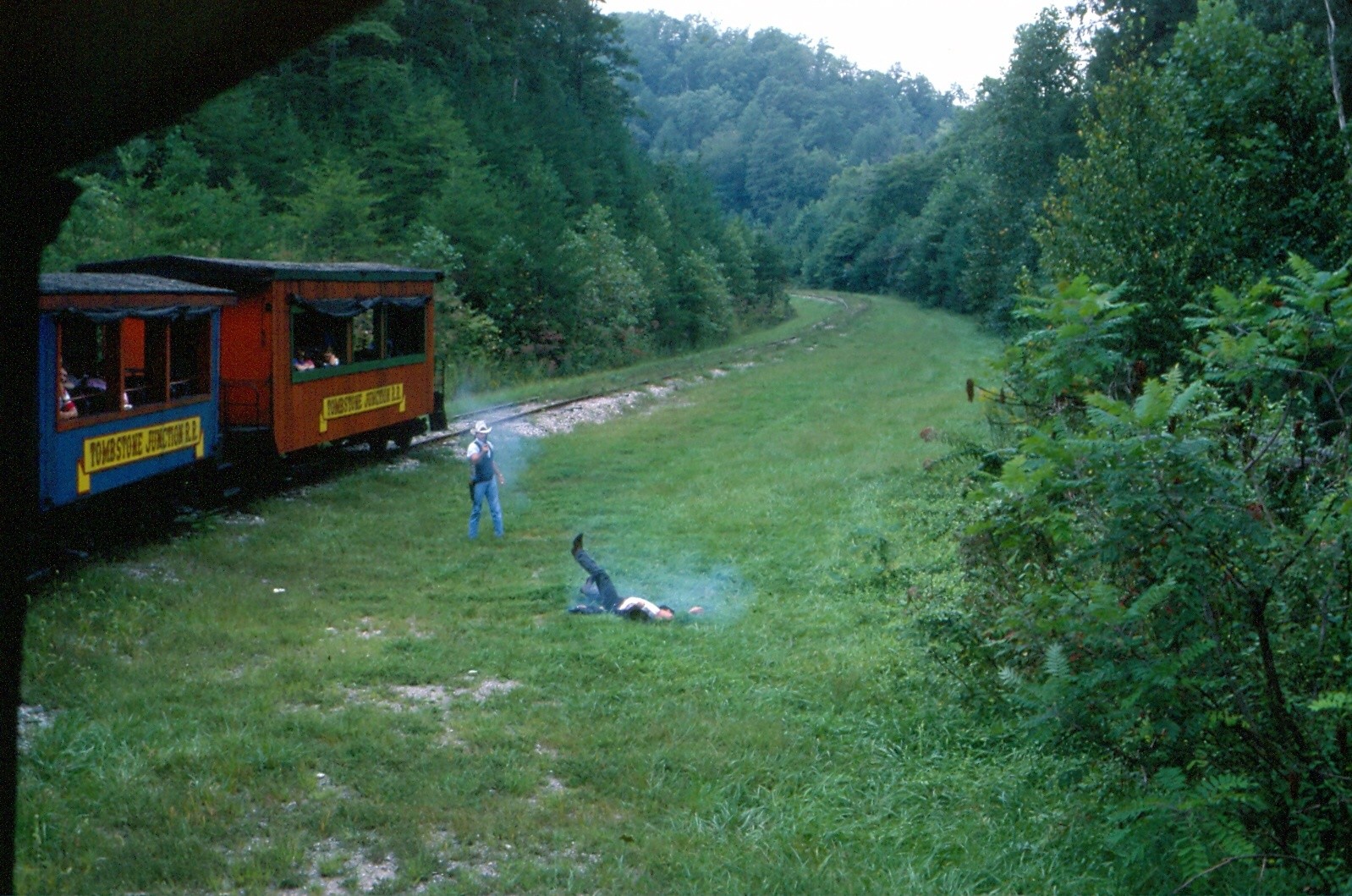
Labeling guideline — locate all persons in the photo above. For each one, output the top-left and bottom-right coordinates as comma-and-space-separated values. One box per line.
571, 531, 705, 624
57, 349, 190, 420
291, 344, 339, 372
465, 420, 507, 540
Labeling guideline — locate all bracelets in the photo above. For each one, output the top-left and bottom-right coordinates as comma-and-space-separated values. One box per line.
497, 473, 503, 478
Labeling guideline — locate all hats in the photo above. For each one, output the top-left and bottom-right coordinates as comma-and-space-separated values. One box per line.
470, 420, 493, 436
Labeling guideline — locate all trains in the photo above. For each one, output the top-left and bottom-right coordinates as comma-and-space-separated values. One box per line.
28, 250, 449, 582
